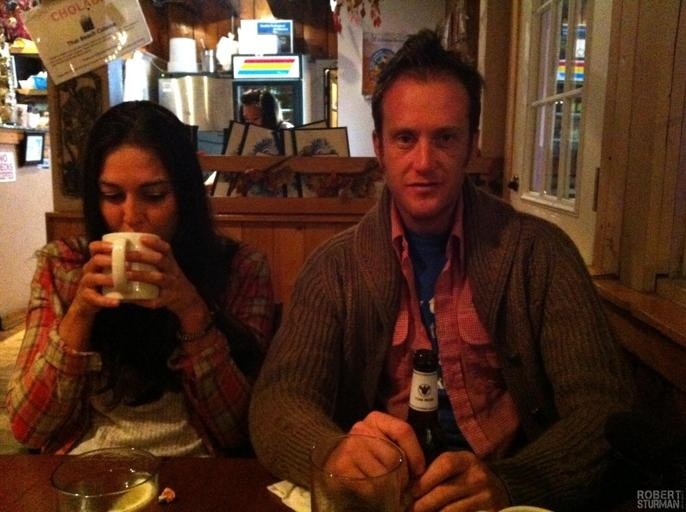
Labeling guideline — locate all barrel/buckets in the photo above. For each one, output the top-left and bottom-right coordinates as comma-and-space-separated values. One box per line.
199, 50, 218, 73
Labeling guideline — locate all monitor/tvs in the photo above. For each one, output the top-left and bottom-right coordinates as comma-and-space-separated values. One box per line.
556, 57, 584, 82
22, 132, 45, 166
231, 55, 302, 80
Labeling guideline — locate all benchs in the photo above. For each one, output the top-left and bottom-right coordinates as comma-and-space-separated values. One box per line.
44, 211, 387, 315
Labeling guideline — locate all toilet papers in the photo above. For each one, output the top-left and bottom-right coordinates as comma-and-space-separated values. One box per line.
169, 37, 199, 72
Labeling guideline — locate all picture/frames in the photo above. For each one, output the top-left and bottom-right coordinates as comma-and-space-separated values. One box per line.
211, 119, 350, 199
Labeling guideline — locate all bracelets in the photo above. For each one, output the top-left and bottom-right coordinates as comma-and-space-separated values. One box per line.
176, 311, 216, 343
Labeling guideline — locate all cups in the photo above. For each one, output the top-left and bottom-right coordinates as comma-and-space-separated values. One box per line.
102, 231, 159, 303
312, 431, 401, 511
50, 446, 158, 511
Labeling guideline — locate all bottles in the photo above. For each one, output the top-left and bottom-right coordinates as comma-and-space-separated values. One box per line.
409, 348, 446, 466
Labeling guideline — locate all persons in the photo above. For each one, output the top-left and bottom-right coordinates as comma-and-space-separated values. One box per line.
238, 87, 295, 130
7, 101, 275, 464
246, 27, 637, 512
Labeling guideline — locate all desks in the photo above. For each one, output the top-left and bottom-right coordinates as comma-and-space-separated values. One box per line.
0, 456, 303, 512
